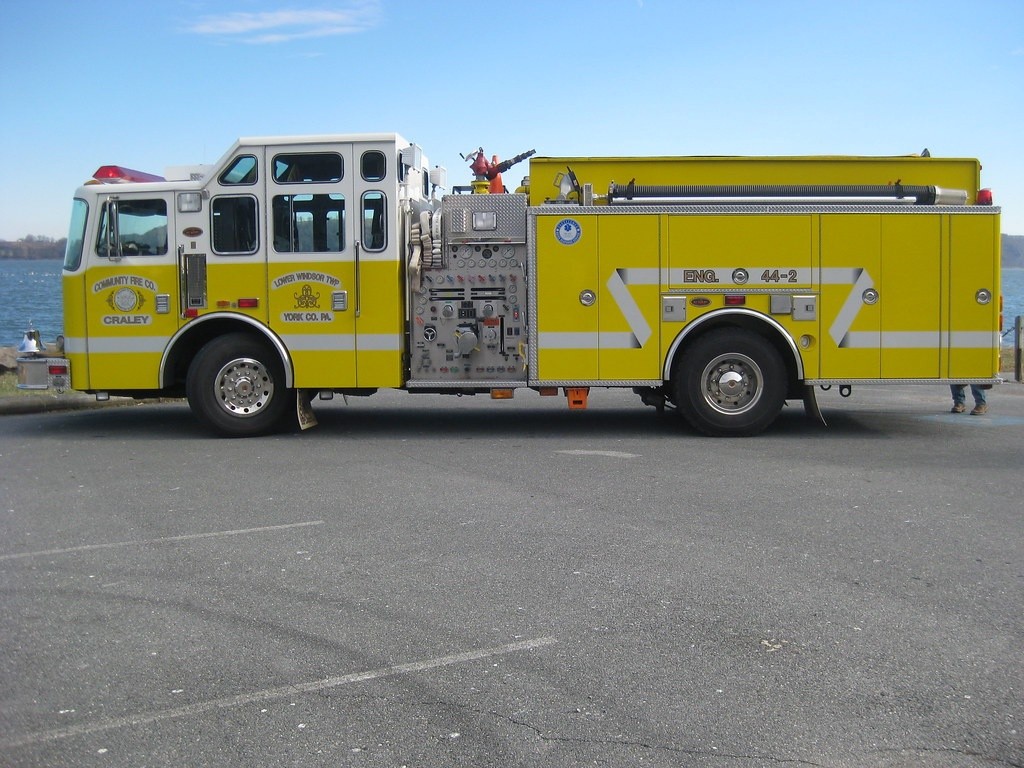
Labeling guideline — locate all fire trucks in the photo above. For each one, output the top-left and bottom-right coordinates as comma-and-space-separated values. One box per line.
10, 133, 1007, 438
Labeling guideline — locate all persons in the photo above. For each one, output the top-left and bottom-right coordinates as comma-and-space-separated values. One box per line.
56, 335, 65, 354
950, 384, 989, 416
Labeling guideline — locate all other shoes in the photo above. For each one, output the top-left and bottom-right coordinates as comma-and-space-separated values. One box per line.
970, 404, 989, 415
951, 401, 966, 413
